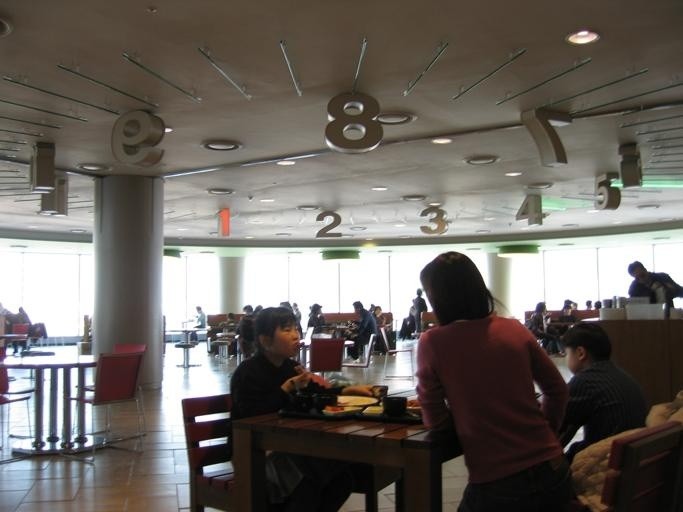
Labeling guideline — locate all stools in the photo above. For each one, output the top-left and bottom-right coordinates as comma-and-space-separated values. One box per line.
175, 325, 241, 369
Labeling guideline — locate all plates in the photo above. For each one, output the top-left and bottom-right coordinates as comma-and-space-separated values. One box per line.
361, 406, 424, 423
322, 405, 364, 418
336, 395, 380, 407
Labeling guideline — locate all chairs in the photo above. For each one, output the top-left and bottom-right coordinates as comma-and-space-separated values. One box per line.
600, 418, 683, 511
297, 324, 439, 381
181, 395, 236, 512
0, 323, 149, 464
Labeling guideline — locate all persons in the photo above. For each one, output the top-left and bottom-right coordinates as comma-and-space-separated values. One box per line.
226, 306, 372, 512
373, 304, 386, 333
350, 301, 373, 365
401, 297, 418, 341
626, 259, 682, 322
306, 304, 327, 336
10, 306, 30, 354
418, 251, 579, 511
191, 306, 205, 342
414, 288, 429, 333
209, 300, 303, 356
527, 298, 603, 357
554, 319, 651, 460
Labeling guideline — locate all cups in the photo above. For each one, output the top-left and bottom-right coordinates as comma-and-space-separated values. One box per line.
371, 385, 388, 400
382, 396, 407, 416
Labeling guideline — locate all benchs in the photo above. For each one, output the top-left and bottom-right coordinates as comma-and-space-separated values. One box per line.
320, 313, 393, 334
525, 310, 600, 324
420, 311, 439, 330
